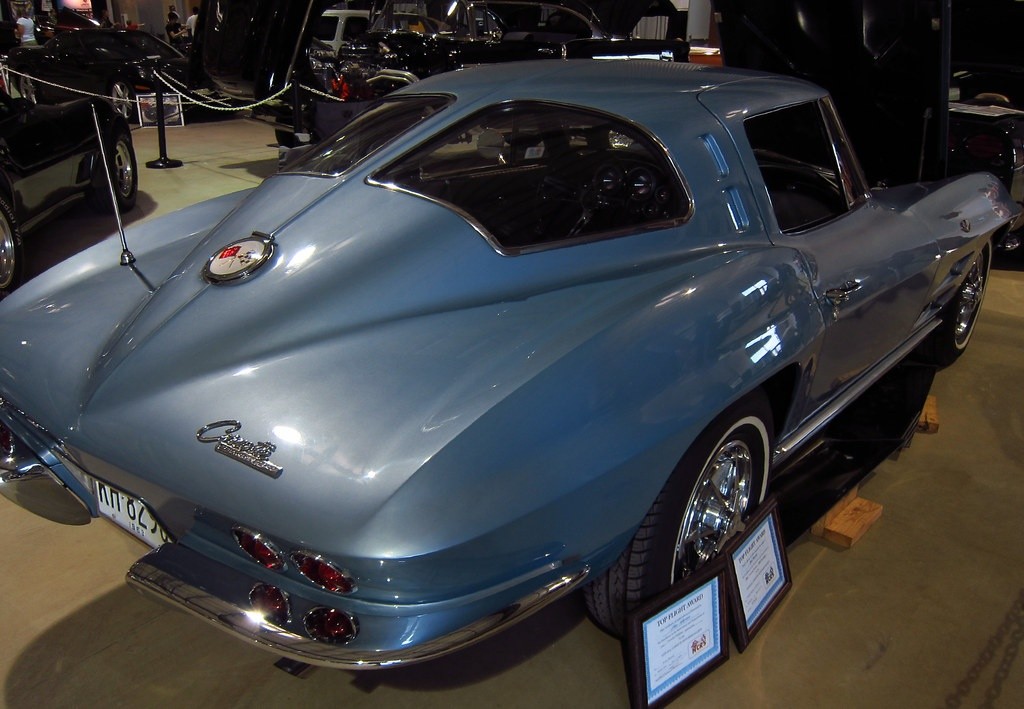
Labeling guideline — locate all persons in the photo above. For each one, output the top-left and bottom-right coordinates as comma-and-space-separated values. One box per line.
168, 6, 181, 24
185, 6, 199, 40
100, 10, 112, 29
13, 7, 39, 47
126, 20, 145, 31
165, 13, 187, 55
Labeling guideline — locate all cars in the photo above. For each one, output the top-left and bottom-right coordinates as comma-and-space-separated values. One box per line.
314, 10, 445, 57
0, 90, 140, 297
3, 26, 215, 122
463, 1, 628, 47
0, 60, 1024, 680
